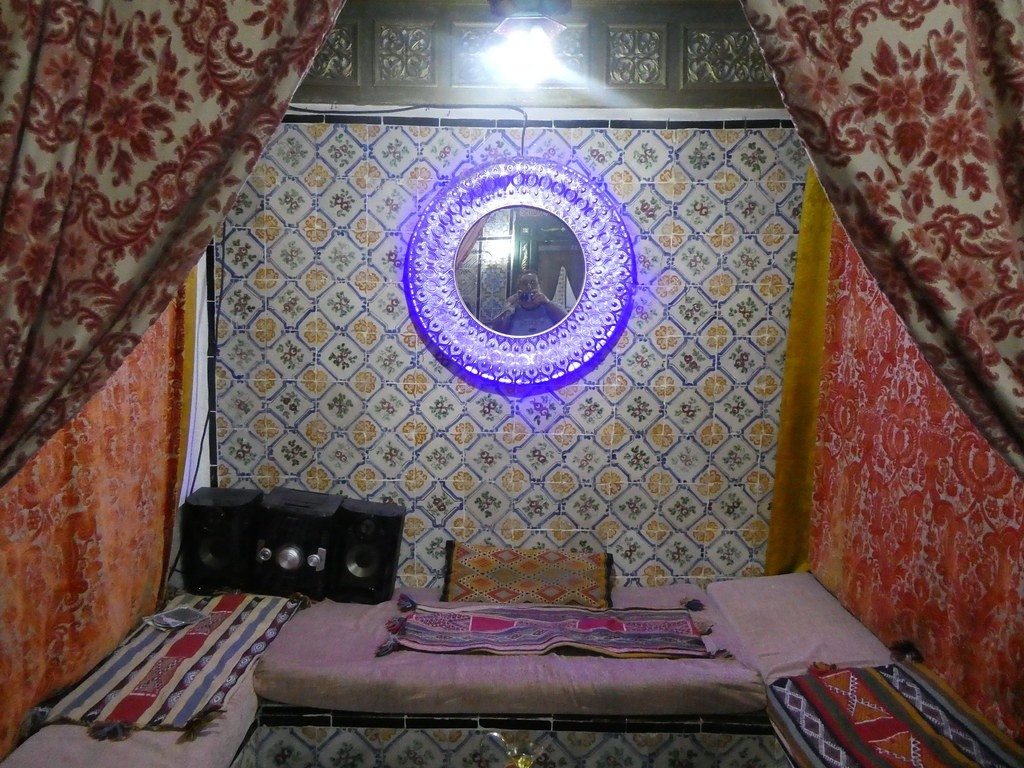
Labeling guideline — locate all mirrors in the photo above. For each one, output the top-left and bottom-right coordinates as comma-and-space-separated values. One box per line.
405, 156, 634, 398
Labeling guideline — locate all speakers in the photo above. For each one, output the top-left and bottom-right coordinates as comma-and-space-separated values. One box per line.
183, 487, 407, 604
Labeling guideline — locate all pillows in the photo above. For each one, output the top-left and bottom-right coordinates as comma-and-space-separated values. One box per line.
440, 540, 613, 609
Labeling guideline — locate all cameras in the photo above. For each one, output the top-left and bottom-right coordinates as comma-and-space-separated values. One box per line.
520, 292, 534, 302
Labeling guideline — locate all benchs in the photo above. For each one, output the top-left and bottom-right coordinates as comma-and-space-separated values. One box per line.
0, 570, 1023, 768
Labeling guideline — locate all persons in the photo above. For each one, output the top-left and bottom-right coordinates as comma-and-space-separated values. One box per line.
493, 270, 568, 336
